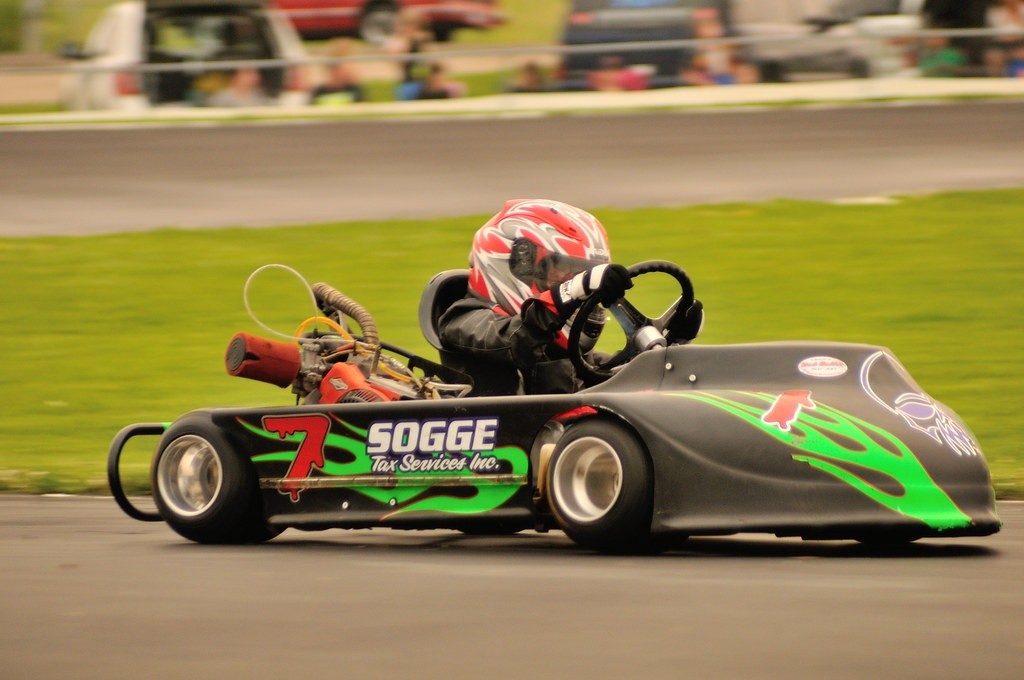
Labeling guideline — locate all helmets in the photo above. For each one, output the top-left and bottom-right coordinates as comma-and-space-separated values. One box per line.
466, 199, 610, 359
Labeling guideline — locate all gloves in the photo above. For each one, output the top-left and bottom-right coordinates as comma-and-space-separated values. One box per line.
666, 299, 704, 345
551, 264, 634, 319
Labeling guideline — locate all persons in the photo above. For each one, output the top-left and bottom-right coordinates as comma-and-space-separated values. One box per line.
438, 199, 704, 398
415, 62, 450, 101
681, 9, 761, 86
207, 68, 266, 108
981, 0, 1024, 77
311, 64, 363, 104
587, 54, 649, 90
921, 37, 968, 78
504, 64, 543, 92
